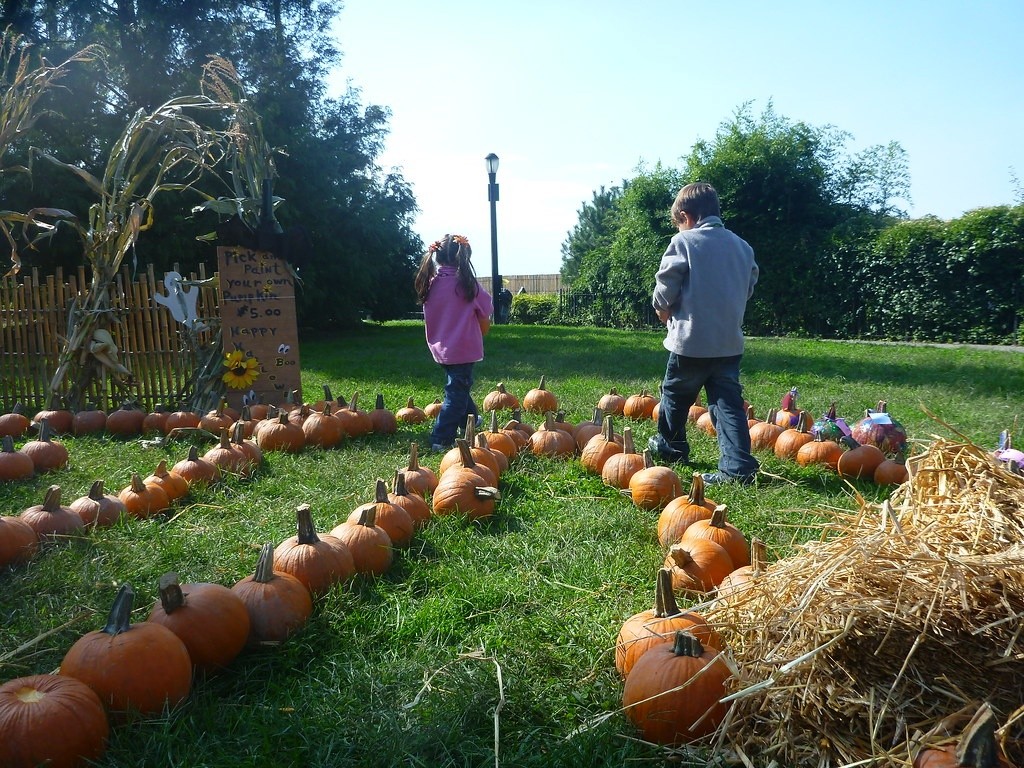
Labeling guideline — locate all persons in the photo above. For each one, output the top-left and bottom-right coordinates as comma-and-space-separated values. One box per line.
647, 182, 760, 487
414, 234, 494, 452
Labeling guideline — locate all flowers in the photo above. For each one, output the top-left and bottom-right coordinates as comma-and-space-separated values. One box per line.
221, 350, 260, 391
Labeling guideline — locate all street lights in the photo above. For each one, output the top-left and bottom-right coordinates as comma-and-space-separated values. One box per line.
485, 153, 501, 324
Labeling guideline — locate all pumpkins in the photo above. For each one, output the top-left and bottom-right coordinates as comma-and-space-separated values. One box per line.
912, 701, 1024, 768
615, 569, 738, 747
600, 387, 923, 486
429, 378, 773, 602
0, 440, 438, 768
478, 319, 490, 334
0, 385, 428, 567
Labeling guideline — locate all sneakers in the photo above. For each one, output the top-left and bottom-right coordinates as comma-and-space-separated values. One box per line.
702, 473, 754, 487
475, 415, 483, 428
648, 433, 688, 464
432, 443, 448, 453
